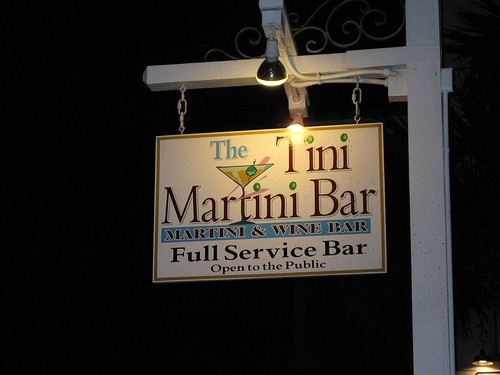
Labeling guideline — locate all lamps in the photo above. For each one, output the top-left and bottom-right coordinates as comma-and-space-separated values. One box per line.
256, 27, 286, 92
